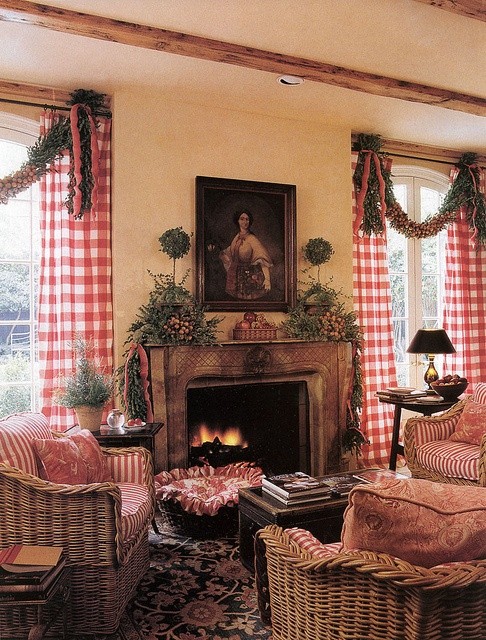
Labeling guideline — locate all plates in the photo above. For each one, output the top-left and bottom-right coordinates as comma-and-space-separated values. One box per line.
124, 422, 147, 430
386, 387, 416, 394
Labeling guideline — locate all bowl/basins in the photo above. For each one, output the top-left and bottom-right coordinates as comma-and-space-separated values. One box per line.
430, 382, 469, 401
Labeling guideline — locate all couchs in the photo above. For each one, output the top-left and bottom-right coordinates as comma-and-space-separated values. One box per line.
403, 382, 486, 487
0, 411, 154, 636
251, 530, 485, 640
154, 460, 263, 538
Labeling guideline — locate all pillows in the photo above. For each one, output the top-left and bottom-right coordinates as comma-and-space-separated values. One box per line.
338, 479, 485, 567
448, 400, 486, 445
69, 427, 109, 480
31, 436, 90, 485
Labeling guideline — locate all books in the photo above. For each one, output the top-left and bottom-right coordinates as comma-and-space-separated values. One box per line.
374, 390, 427, 402
0, 545, 67, 602
320, 476, 369, 497
261, 472, 332, 506
353, 470, 409, 485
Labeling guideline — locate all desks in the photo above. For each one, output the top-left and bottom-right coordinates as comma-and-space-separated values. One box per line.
63, 422, 168, 472
237, 466, 410, 573
0, 567, 73, 640
377, 392, 464, 471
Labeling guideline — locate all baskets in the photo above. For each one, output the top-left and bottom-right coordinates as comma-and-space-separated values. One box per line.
154, 488, 262, 538
233, 328, 277, 341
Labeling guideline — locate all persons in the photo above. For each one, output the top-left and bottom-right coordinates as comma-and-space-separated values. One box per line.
208, 209, 275, 300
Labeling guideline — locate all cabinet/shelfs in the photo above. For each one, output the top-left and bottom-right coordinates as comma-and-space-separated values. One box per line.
143, 336, 354, 476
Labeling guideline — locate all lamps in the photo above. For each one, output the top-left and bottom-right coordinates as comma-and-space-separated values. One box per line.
406, 327, 456, 394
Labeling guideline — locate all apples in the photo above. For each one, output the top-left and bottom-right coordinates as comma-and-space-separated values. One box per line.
235, 320, 242, 329
239, 320, 251, 330
244, 312, 257, 322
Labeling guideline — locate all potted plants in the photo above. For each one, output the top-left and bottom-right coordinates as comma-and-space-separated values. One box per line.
297, 236, 338, 315
47, 330, 119, 434
147, 224, 192, 318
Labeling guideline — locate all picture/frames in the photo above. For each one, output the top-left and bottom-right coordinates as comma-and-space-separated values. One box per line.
193, 175, 298, 314
